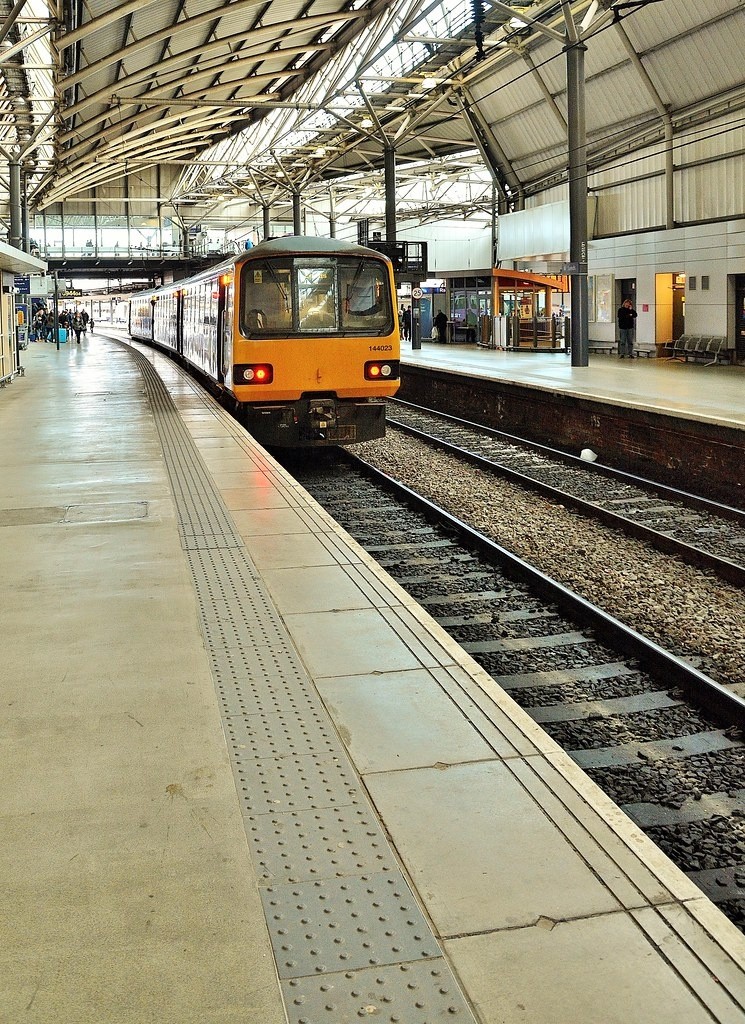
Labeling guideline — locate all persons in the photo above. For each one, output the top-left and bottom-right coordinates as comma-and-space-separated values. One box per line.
616, 300, 637, 359
464, 308, 478, 330
23, 232, 257, 257
434, 308, 449, 342
300, 308, 325, 329
399, 302, 412, 341
32, 297, 96, 343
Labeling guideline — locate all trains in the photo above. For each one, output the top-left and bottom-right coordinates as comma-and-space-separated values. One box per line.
126, 235, 405, 452
